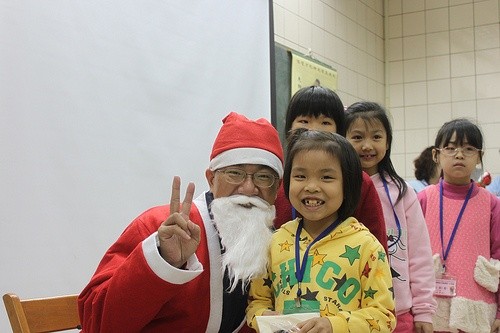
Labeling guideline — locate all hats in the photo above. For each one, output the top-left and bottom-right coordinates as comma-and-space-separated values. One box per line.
209, 112, 285, 180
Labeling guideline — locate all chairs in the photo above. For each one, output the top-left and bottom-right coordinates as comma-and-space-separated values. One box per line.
3, 291, 83, 333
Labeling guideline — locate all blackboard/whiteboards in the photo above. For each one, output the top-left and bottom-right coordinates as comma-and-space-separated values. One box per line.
272, 41, 338, 168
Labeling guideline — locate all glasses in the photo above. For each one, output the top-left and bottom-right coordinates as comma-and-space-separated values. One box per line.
214, 167, 280, 188
436, 146, 482, 156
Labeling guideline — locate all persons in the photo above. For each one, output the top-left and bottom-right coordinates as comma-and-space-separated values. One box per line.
416, 118, 500, 333
341, 101, 436, 332
246, 128, 398, 333
405, 146, 443, 194
273, 85, 389, 266
79, 111, 286, 333
